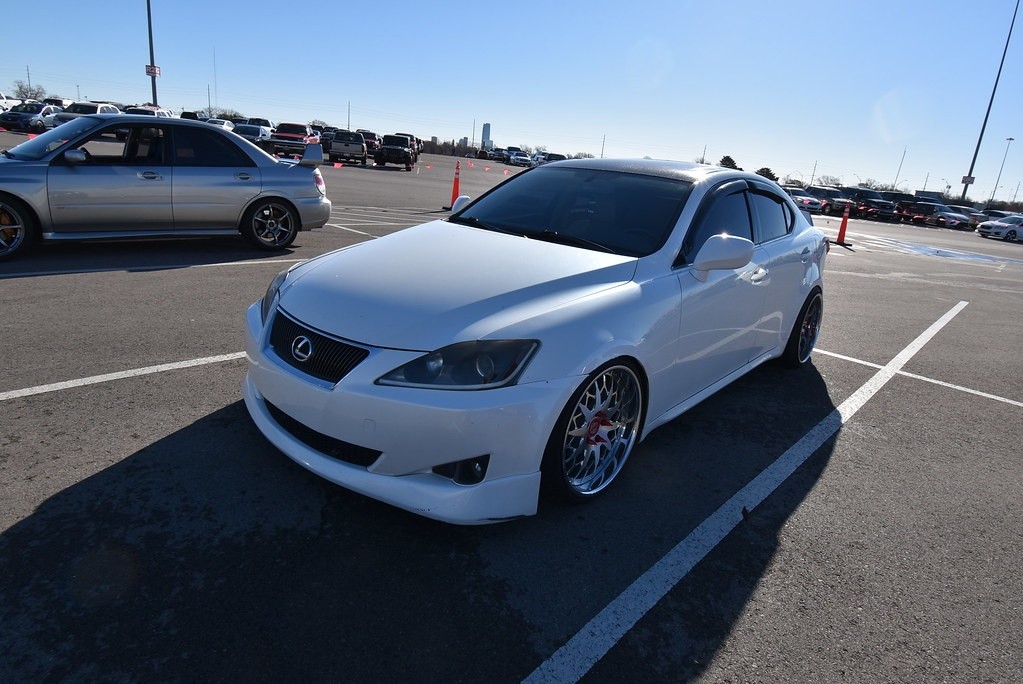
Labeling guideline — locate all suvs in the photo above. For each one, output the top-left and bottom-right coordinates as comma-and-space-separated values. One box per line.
805, 186, 856, 218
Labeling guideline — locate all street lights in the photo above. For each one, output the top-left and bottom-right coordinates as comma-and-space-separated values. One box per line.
990, 138, 1015, 203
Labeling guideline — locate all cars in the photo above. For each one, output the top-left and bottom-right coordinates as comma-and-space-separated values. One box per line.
781, 186, 823, 215
838, 186, 1023, 231
0, 92, 425, 172
465, 146, 568, 168
974, 216, 1023, 242
0, 113, 330, 266
240, 156, 832, 527
